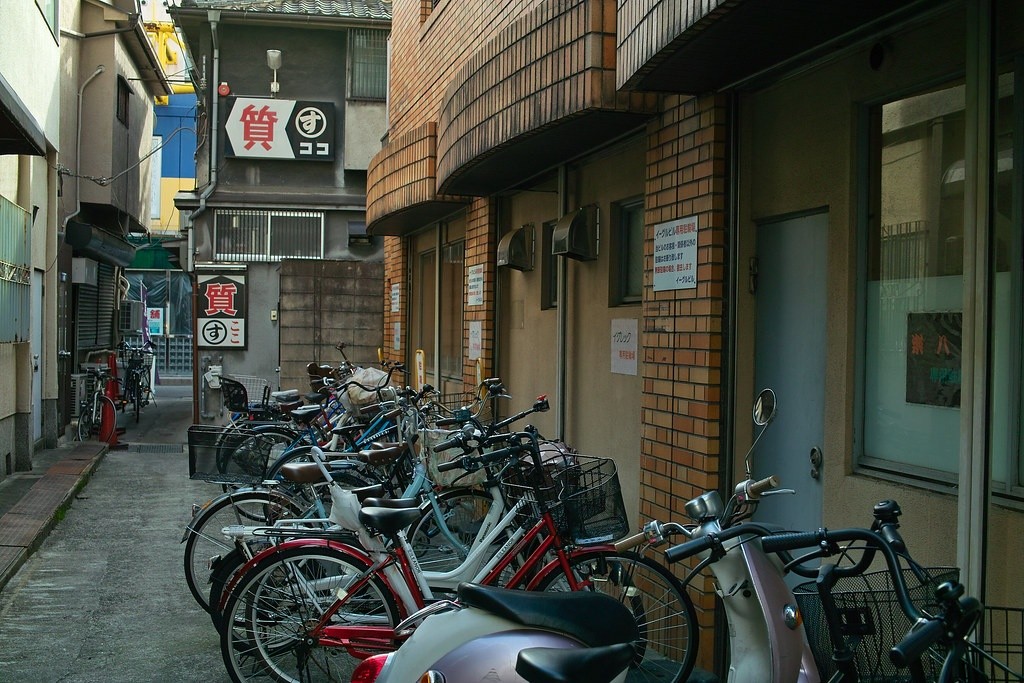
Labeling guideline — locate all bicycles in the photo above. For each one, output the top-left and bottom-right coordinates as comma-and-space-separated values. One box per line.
119, 347, 155, 423
178, 341, 700, 683
117, 340, 157, 408
78, 366, 123, 442
514, 499, 907, 683
761, 523, 1024, 683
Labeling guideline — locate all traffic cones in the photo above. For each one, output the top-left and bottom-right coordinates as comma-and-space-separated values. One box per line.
98, 377, 129, 448
108, 354, 126, 404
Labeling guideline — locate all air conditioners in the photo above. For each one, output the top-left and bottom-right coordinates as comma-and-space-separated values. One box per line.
67, 374, 88, 416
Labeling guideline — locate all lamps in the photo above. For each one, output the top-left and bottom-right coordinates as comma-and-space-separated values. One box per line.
264, 49, 282, 93
552, 205, 600, 263
348, 221, 374, 246
497, 224, 535, 271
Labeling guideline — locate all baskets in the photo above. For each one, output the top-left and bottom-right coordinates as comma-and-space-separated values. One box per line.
501, 453, 606, 534
420, 391, 494, 434
793, 566, 962, 683
959, 607, 1024, 683
553, 458, 630, 546
228, 373, 268, 405
187, 425, 276, 485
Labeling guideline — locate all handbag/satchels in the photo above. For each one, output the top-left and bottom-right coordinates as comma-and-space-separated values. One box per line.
338, 367, 394, 413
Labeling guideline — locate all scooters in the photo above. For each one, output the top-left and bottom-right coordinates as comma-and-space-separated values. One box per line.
350, 386, 820, 683
